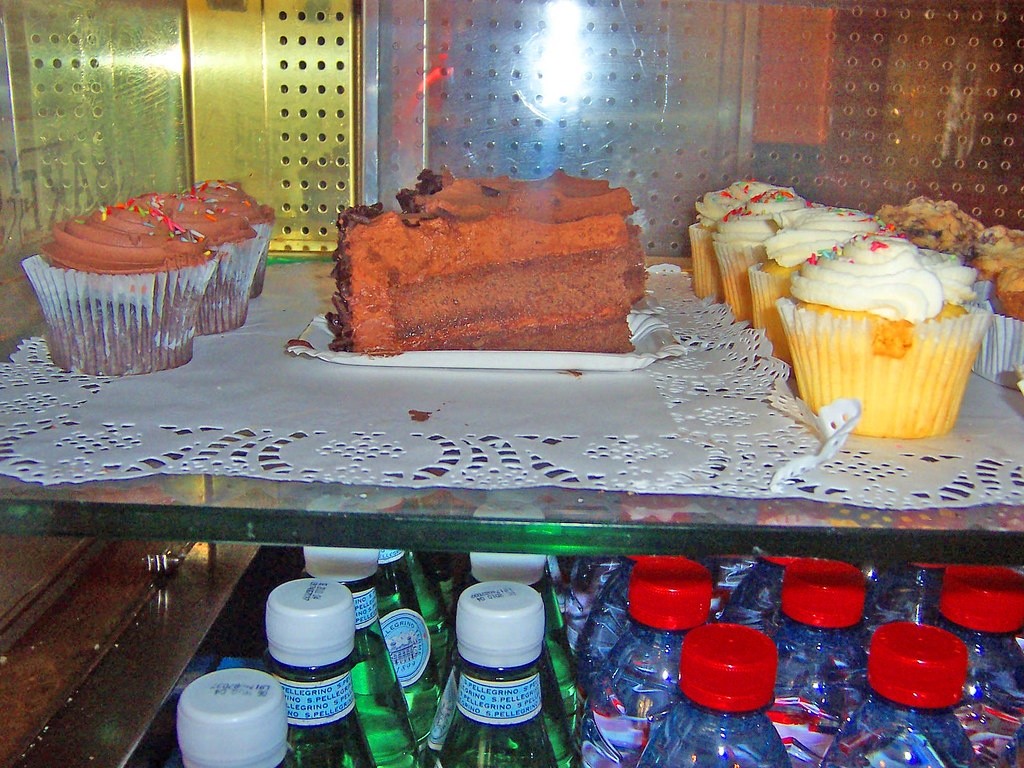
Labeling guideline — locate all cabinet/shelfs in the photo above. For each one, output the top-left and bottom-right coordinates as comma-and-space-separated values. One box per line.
0, 0, 1024, 766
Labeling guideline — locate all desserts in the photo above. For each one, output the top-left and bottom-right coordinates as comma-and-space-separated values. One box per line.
21, 168, 1024, 438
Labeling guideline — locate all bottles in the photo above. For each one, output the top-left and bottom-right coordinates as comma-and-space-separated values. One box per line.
174, 494, 1023, 768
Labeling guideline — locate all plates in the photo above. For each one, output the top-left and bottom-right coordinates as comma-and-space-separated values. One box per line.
283, 315, 690, 372
